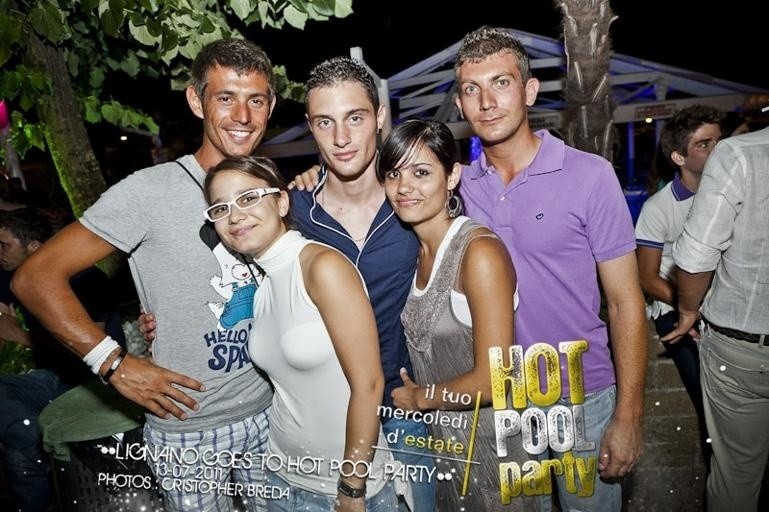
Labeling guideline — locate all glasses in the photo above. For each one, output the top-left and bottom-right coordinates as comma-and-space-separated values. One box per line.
202, 188, 281, 223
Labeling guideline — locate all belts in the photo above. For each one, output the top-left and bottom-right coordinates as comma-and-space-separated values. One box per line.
700, 315, 769, 346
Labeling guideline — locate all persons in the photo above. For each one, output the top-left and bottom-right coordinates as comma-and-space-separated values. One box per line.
0, 27, 769, 512
